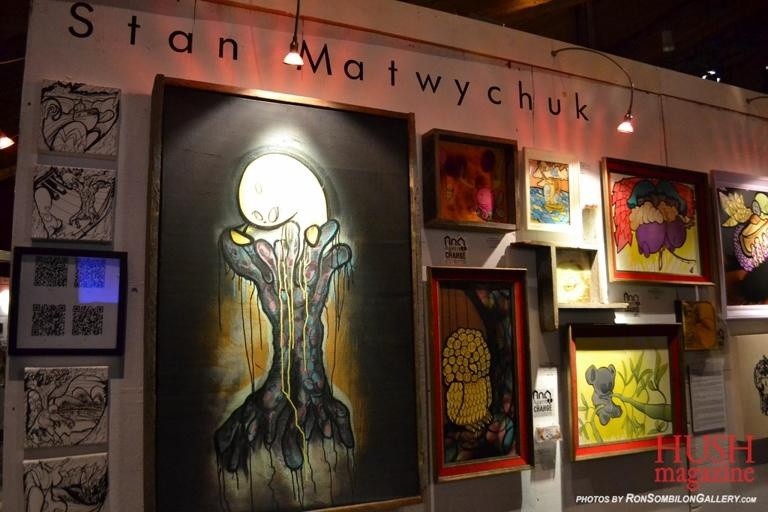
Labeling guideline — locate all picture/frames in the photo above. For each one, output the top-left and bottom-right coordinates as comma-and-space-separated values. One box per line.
601, 155, 712, 285
562, 323, 683, 462
142, 73, 428, 512
522, 144, 578, 233
681, 299, 717, 352
422, 127, 522, 233
7, 245, 128, 358
708, 167, 768, 315
426, 265, 536, 485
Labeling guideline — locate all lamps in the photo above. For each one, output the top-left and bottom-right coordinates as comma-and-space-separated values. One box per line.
283, 3, 307, 68
551, 45, 637, 136
